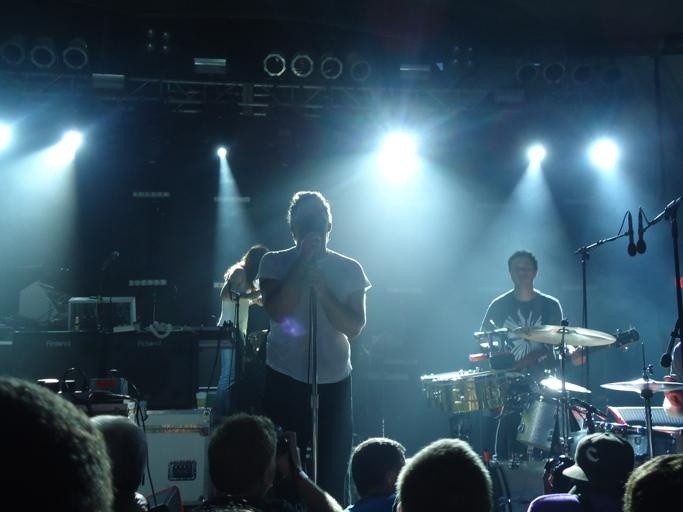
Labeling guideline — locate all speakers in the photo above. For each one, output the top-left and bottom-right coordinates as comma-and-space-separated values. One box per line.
106, 327, 232, 410
12, 328, 105, 390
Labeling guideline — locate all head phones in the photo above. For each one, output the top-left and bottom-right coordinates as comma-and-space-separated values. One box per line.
60, 366, 97, 403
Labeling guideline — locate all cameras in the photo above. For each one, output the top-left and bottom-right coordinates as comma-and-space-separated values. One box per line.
274, 428, 289, 455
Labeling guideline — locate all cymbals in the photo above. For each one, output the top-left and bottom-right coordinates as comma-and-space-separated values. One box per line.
541, 377, 591, 394
598, 378, 682, 393
511, 324, 616, 347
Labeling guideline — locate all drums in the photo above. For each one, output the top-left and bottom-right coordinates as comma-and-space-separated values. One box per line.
591, 422, 682, 464
515, 393, 558, 452
419, 371, 507, 414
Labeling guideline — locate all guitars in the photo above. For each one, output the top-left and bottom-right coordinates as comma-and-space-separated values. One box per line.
513, 329, 639, 367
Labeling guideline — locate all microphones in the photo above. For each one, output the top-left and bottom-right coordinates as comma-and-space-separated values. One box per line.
627, 214, 635, 254
660, 317, 682, 367
636, 208, 647, 253
571, 397, 608, 420
473, 327, 508, 340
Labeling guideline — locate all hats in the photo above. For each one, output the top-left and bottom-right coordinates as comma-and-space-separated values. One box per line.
561, 430, 636, 487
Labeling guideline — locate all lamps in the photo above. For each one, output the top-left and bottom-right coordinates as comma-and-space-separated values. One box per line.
0, 26, 594, 91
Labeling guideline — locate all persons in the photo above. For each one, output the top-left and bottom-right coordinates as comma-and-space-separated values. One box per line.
247, 191, 373, 503
214, 244, 269, 411
482, 250, 586, 462
